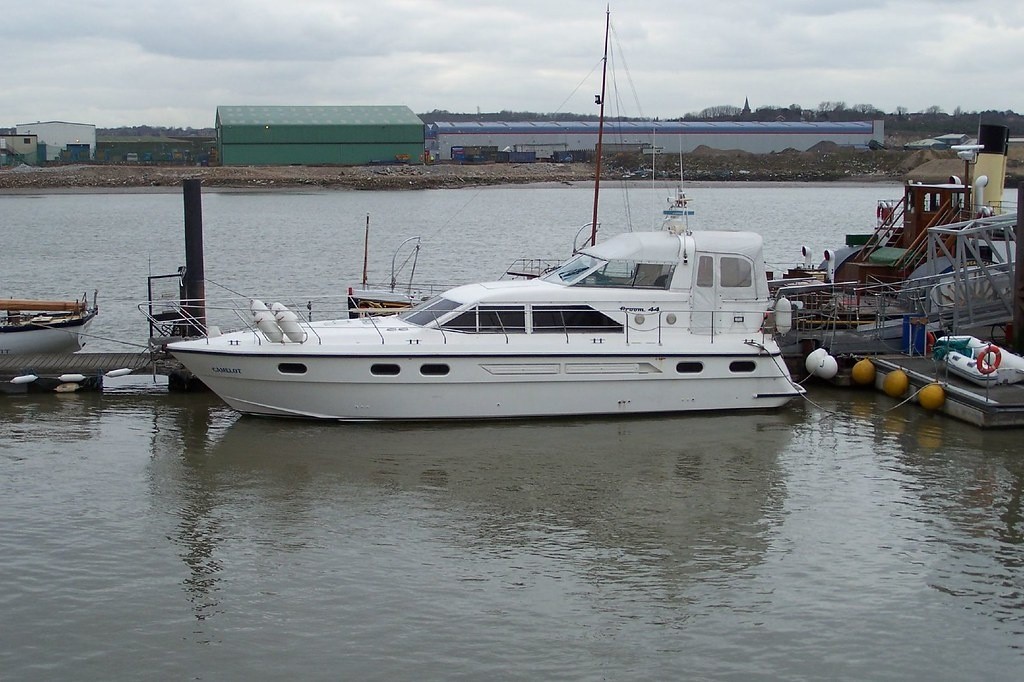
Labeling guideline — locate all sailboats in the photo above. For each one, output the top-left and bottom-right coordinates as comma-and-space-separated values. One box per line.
505, 2, 662, 280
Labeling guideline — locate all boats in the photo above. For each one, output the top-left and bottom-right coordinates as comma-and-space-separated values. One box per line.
346, 209, 467, 320
772, 122, 1024, 433
138, 125, 810, 414
0, 291, 101, 353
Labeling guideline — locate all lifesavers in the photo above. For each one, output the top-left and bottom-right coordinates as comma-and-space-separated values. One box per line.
976, 205, 994, 227
977, 346, 1002, 374
877, 201, 894, 220
